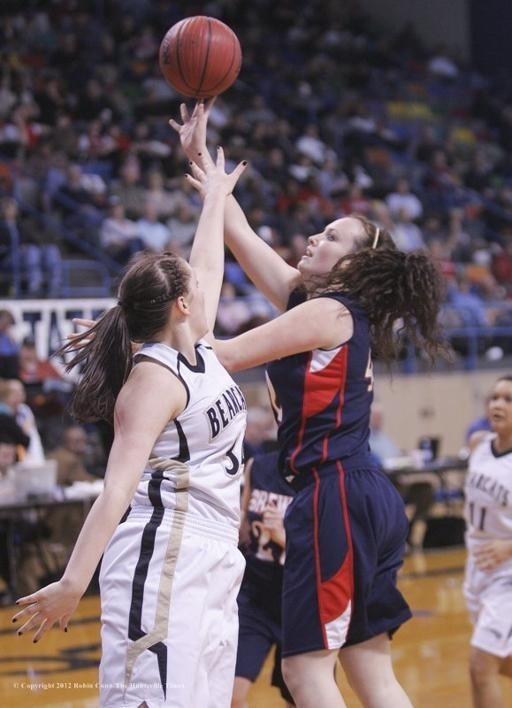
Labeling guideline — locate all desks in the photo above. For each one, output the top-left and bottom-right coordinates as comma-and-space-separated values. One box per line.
0, 459, 470, 602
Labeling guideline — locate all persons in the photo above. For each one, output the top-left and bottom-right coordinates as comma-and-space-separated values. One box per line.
229, 445, 340, 708
458, 373, 510, 706
0, 1, 511, 602
66, 94, 444, 708
9, 143, 250, 708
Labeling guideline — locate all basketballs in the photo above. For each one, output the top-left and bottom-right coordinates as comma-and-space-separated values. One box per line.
159, 15, 241, 99
